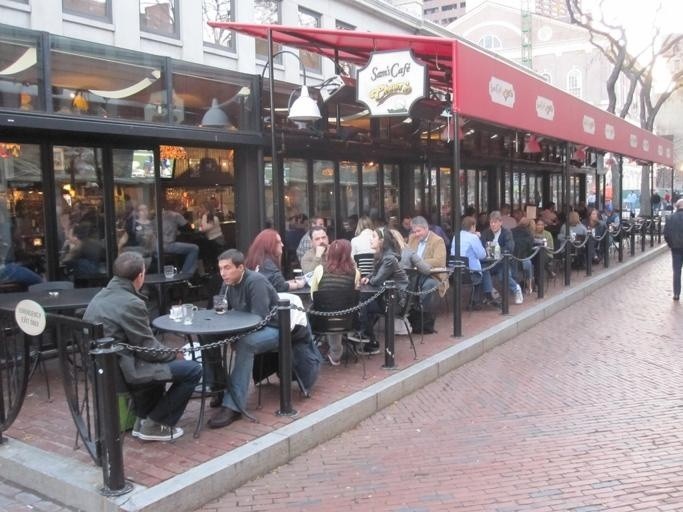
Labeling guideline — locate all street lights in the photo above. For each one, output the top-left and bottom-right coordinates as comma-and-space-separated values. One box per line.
252, 51, 323, 267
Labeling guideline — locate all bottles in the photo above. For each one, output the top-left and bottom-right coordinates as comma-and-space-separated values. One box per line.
486, 241, 492, 258
494, 242, 501, 259
572, 231, 576, 241
587, 226, 596, 237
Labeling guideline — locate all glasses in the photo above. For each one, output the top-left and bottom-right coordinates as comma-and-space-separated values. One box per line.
378, 226, 385, 240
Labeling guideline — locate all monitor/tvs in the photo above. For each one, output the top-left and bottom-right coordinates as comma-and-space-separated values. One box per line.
131, 149, 176, 179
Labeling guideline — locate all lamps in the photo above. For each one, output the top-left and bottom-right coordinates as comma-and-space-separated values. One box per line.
441, 114, 465, 142
571, 145, 585, 160
524, 135, 542, 152
606, 152, 618, 165
261, 50, 323, 122
201, 96, 230, 127
440, 72, 453, 118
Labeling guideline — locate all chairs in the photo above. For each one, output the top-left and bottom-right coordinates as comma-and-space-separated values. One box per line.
354, 254, 382, 279
27, 282, 77, 292
446, 256, 484, 311
310, 289, 367, 380
368, 267, 419, 364
228, 292, 322, 409
72, 331, 180, 450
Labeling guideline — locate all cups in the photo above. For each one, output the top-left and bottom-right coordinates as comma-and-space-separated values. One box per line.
170, 304, 198, 325
293, 269, 303, 278
543, 238, 547, 247
163, 265, 177, 278
295, 276, 305, 289
213, 295, 228, 314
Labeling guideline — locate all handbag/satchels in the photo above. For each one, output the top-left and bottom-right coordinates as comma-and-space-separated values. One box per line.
407, 309, 435, 334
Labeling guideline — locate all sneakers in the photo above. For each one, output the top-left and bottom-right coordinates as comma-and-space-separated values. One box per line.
347, 331, 370, 343
132, 416, 142, 437
139, 421, 184, 441
514, 284, 523, 304
357, 341, 380, 355
482, 288, 500, 304
327, 351, 341, 366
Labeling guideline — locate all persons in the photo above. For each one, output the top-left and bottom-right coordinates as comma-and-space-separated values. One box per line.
287, 212, 450, 366
197, 248, 280, 428
0, 196, 225, 287
82, 251, 202, 440
663, 199, 683, 300
653, 192, 660, 216
450, 201, 620, 304
244, 228, 307, 290
627, 191, 637, 218
663, 193, 671, 206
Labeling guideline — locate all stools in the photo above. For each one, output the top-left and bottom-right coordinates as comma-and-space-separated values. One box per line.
154, 251, 179, 271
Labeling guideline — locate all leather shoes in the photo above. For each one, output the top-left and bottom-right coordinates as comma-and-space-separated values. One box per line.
207, 407, 241, 428
210, 390, 224, 407
